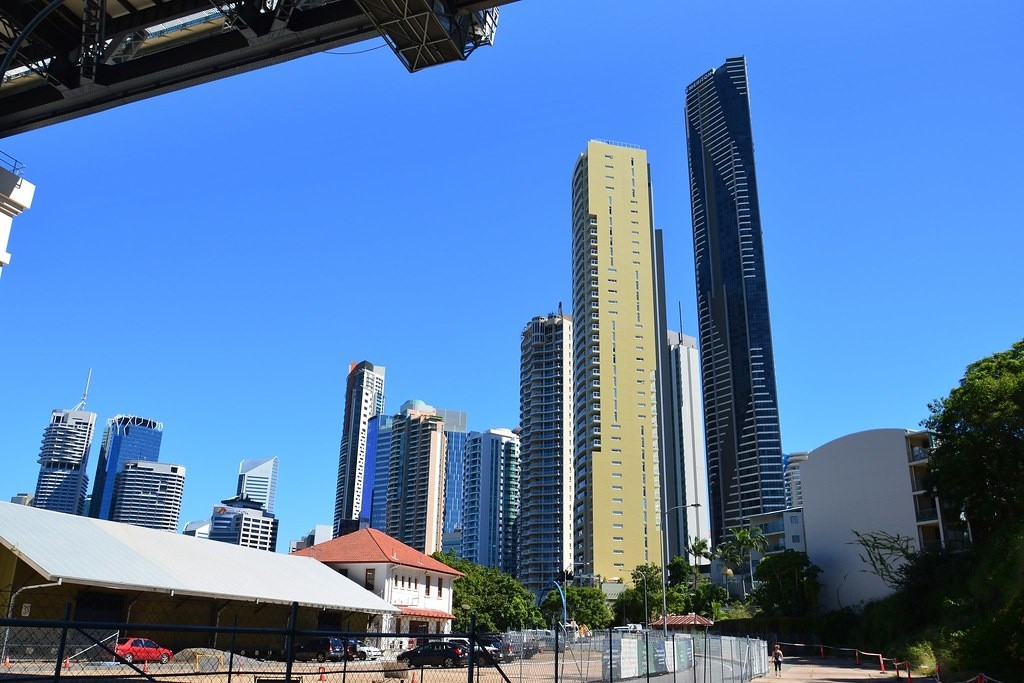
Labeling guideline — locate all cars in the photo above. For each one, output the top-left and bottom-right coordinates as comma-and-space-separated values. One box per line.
397, 642, 469, 669
471, 629, 569, 663
98, 637, 173, 664
341, 637, 382, 661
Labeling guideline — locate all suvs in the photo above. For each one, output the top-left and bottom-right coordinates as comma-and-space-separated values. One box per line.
291, 635, 360, 663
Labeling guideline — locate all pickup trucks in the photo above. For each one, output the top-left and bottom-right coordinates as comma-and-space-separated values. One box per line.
416, 638, 502, 667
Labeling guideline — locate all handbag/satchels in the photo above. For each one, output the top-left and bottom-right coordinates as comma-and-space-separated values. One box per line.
778, 656, 782, 661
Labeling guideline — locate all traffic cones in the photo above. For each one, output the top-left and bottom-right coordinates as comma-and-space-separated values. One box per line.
411, 672, 417, 682
318, 673, 327, 681
237, 664, 245, 677
63, 656, 71, 668
139, 659, 150, 675
320, 667, 326, 673
3, 655, 12, 668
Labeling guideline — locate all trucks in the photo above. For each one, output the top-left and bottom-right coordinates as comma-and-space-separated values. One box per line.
562, 621, 590, 642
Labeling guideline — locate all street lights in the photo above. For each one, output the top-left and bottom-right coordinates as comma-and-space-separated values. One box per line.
564, 562, 590, 643
618, 569, 648, 630
660, 503, 701, 637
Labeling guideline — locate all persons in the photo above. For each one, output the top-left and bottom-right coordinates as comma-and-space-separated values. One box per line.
771, 645, 783, 677
